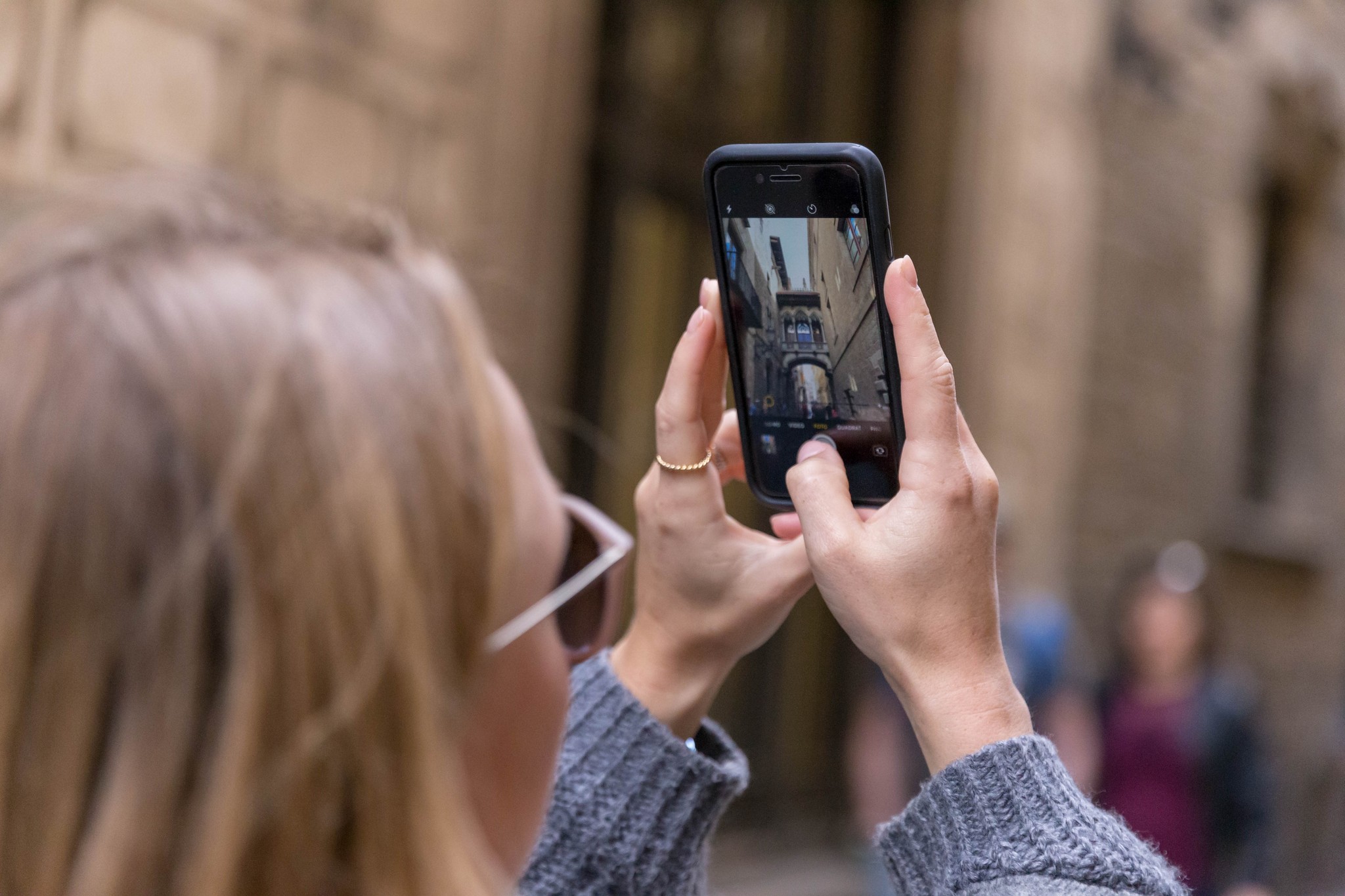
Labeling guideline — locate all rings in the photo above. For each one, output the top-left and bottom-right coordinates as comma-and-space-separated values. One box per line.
654, 453, 718, 475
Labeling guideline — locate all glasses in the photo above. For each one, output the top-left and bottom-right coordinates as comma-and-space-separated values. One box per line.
475, 484, 631, 666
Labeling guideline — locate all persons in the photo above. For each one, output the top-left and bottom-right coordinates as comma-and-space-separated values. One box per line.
844, 530, 1277, 896
0, 198, 1187, 896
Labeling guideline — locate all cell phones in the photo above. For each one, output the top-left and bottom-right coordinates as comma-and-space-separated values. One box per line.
704, 142, 908, 509
